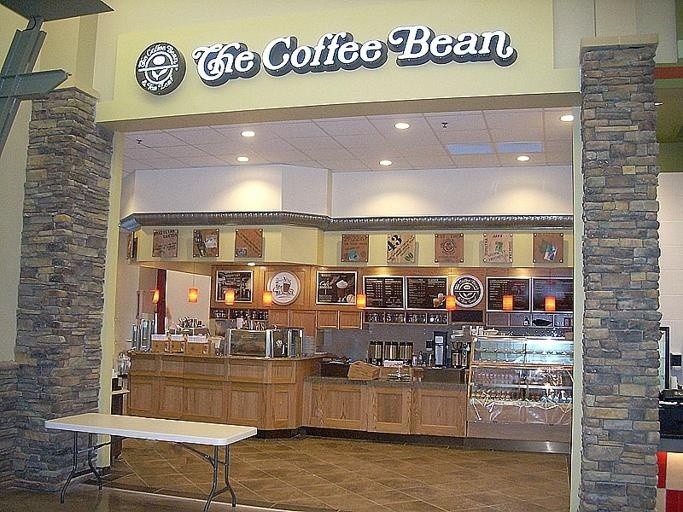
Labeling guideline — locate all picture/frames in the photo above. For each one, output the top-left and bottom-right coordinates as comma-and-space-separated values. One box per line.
658, 327, 670, 389
405, 275, 449, 311
266, 270, 301, 306
362, 275, 405, 310
316, 270, 358, 305
214, 270, 254, 303
531, 276, 574, 314
485, 276, 531, 313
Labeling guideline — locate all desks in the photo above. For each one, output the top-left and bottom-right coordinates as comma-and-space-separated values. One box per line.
43, 412, 259, 512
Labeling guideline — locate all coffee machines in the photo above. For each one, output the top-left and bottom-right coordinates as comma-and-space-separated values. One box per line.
451, 329, 470, 369
425, 331, 448, 368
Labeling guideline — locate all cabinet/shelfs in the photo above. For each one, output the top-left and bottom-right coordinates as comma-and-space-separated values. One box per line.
466, 334, 573, 443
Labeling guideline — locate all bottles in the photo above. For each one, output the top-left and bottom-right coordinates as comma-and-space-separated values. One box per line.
233, 310, 266, 321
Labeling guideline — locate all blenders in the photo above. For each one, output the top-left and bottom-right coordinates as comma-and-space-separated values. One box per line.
368, 341, 412, 367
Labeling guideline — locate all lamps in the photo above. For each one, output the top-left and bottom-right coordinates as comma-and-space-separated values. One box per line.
356, 294, 366, 309
262, 290, 273, 307
446, 295, 456, 311
544, 296, 555, 311
224, 288, 234, 306
149, 288, 159, 304
502, 295, 513, 312
188, 262, 198, 303
